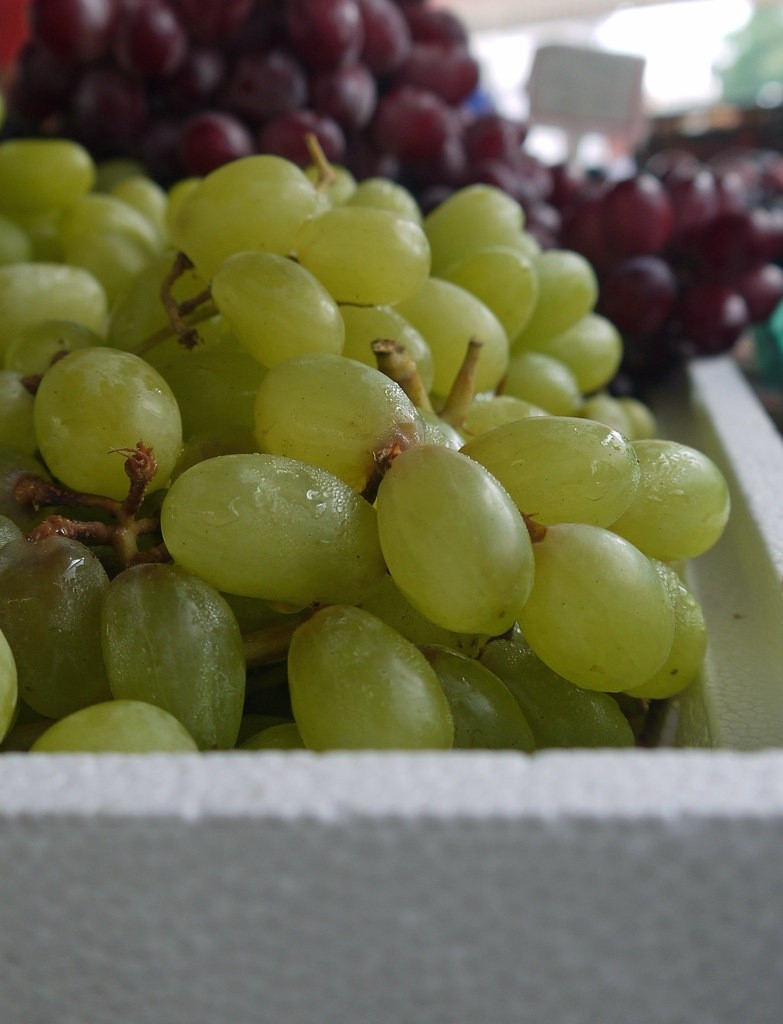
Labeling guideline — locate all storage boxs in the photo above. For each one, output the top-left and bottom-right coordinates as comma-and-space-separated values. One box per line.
0, 350, 783, 1024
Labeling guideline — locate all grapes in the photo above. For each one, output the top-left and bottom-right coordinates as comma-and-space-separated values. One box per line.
0, 0, 783, 756
